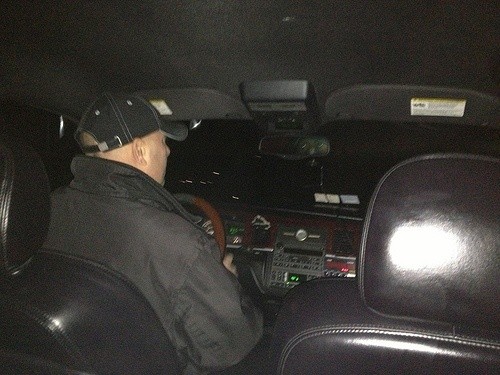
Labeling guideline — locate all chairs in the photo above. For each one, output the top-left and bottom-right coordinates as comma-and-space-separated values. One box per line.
269, 151, 500, 374
0, 132, 181, 374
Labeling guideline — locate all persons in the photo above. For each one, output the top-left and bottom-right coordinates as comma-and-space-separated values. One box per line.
43, 91, 264, 375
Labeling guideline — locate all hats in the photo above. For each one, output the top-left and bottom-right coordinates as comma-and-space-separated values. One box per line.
74, 91, 189, 154
